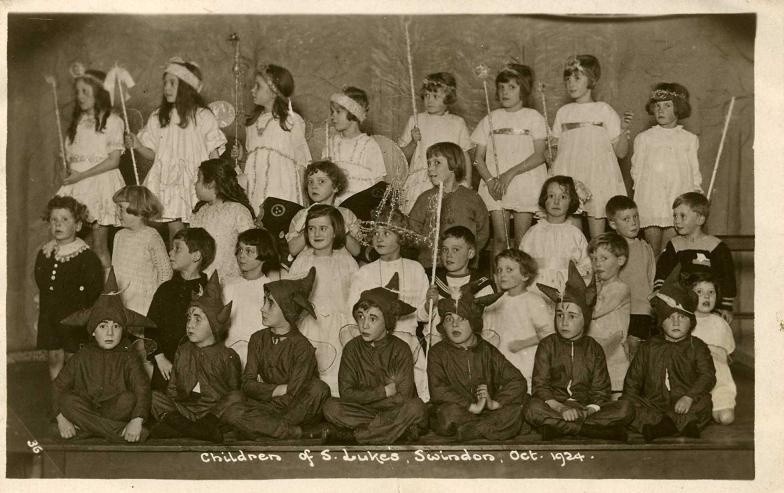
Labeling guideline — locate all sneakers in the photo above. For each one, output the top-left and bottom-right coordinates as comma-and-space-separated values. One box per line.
607, 421, 628, 443
642, 424, 661, 443
203, 422, 222, 443
148, 421, 178, 437
542, 423, 563, 441
321, 425, 352, 445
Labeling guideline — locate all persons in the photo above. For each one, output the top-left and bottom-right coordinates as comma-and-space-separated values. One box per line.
34, 55, 737, 443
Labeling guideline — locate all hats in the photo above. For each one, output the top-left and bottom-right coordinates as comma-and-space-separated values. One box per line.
262, 266, 317, 329
58, 268, 158, 337
537, 261, 598, 338
187, 269, 234, 338
649, 264, 698, 332
437, 281, 502, 332
354, 273, 416, 334
